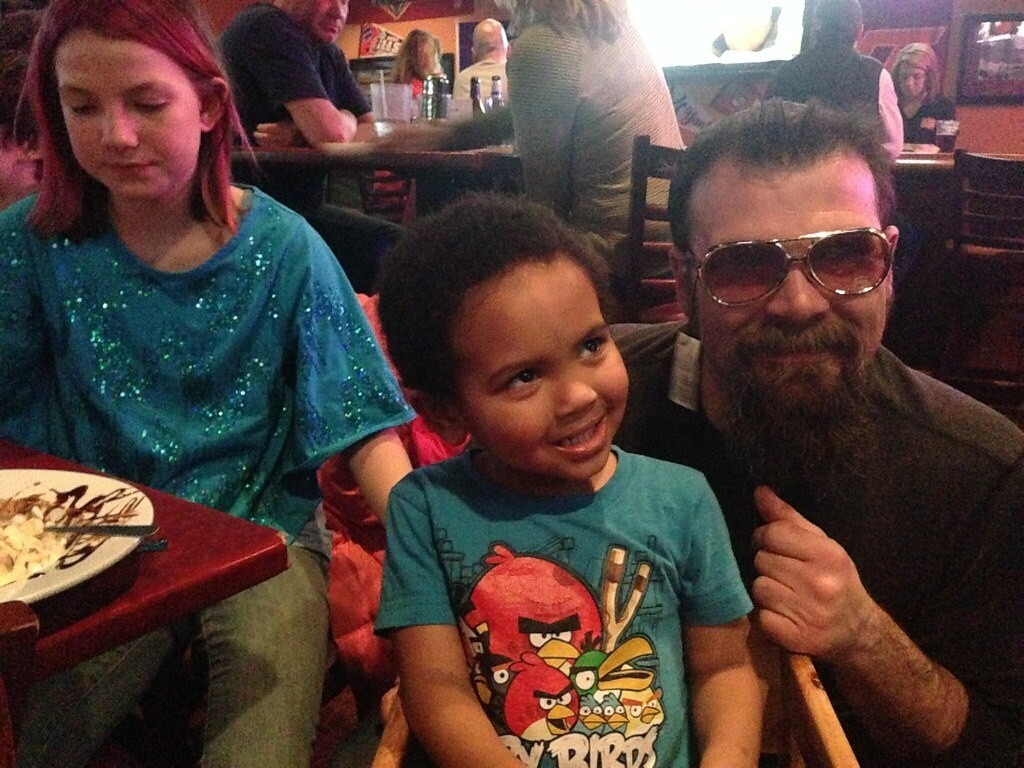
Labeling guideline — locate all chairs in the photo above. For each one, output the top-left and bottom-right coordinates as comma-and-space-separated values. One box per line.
627, 133, 726, 475
356, 165, 409, 223
952, 145, 1024, 427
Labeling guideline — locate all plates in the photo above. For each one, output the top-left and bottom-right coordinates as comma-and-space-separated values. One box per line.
0, 468, 154, 604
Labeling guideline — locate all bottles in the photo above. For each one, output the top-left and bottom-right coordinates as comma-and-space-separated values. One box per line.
491, 75, 504, 109
421, 74, 450, 120
469, 75, 487, 120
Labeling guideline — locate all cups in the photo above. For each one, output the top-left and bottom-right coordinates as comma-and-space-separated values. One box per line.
934, 119, 961, 151
485, 95, 493, 112
368, 82, 422, 138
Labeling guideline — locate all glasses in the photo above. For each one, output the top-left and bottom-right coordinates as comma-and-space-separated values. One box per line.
690, 227, 890, 307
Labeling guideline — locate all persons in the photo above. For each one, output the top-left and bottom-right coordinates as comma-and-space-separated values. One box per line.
765, 0, 903, 157
218, 0, 407, 296
0, 0, 412, 768
608, 102, 1024, 767
450, 19, 512, 153
0, 10, 49, 212
380, 0, 687, 316
394, 28, 442, 99
376, 196, 762, 768
891, 43, 957, 142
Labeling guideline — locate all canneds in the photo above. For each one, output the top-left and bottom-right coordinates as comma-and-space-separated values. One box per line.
421, 73, 449, 122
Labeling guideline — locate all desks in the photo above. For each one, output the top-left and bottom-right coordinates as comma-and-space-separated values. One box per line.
0, 431, 292, 768
891, 152, 1024, 367
286, 144, 523, 184
